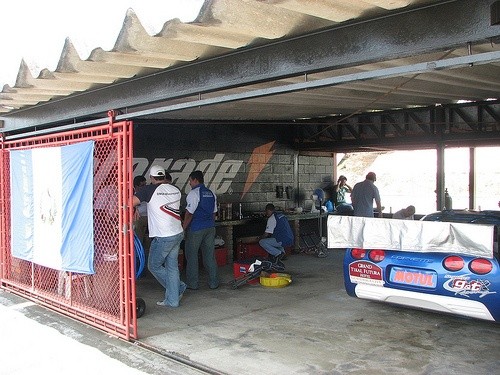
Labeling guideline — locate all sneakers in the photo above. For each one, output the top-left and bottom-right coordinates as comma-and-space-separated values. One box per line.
157, 299, 180, 306
179, 280, 187, 302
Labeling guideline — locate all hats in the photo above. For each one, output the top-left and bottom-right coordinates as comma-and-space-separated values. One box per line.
149, 165, 166, 177
366, 172, 376, 182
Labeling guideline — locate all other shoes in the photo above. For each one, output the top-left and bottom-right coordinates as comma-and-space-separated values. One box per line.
275, 252, 286, 261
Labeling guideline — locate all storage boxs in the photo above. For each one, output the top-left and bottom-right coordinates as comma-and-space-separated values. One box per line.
237, 236, 270, 259
213, 248, 227, 267
233, 263, 260, 284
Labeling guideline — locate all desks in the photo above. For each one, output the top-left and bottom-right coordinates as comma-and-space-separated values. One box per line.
328, 210, 382, 217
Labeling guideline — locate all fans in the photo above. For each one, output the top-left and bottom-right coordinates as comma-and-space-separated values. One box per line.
310, 188, 325, 212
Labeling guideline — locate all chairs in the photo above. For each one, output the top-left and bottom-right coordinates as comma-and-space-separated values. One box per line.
57, 270, 90, 300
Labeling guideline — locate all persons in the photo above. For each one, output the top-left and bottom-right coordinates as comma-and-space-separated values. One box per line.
392, 206, 415, 220
182, 170, 220, 289
94, 178, 119, 256
351, 172, 383, 218
132, 175, 154, 278
259, 203, 294, 262
127, 165, 188, 307
165, 171, 200, 277
335, 175, 384, 217
